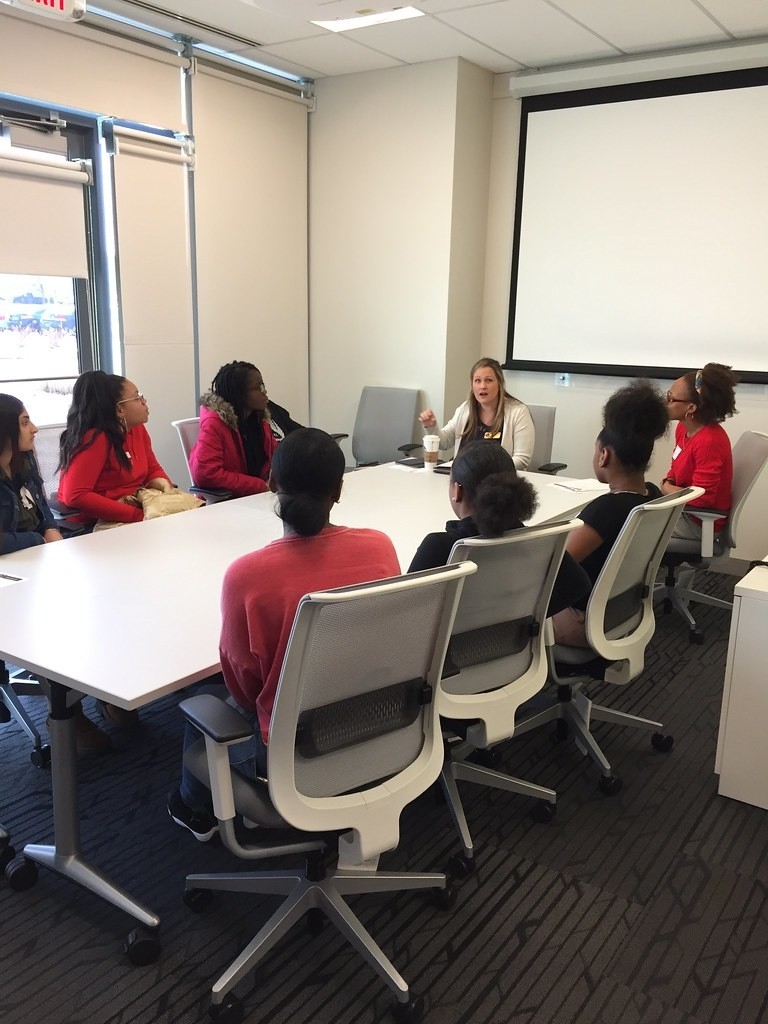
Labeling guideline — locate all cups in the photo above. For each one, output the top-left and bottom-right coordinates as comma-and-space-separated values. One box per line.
423, 435, 440, 469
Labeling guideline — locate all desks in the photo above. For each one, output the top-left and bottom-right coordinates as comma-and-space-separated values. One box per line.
0, 458, 612, 967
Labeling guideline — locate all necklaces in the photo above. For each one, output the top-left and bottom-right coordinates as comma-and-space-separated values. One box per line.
683, 424, 703, 452
614, 489, 648, 496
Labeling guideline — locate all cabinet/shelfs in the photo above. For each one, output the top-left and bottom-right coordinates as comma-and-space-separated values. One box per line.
715, 554, 768, 810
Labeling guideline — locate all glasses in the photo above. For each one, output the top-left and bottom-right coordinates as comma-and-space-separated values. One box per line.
247, 381, 266, 393
666, 390, 701, 410
116, 392, 146, 405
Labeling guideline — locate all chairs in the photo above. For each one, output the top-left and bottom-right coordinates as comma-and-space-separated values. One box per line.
526, 405, 568, 475
440, 519, 584, 870
476, 486, 706, 797
171, 416, 235, 502
653, 428, 768, 644
177, 562, 477, 1024
329, 387, 421, 472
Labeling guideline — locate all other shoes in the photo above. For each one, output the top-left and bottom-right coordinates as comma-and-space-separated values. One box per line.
95, 698, 140, 730
46, 702, 112, 754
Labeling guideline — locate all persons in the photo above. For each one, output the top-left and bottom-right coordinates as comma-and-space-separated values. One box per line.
418, 358, 536, 471
0, 393, 139, 755
659, 362, 741, 539
406, 440, 592, 619
552, 378, 670, 649
53, 371, 174, 539
167, 426, 401, 842
188, 361, 306, 506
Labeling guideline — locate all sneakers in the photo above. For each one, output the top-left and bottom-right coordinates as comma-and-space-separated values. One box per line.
214, 812, 295, 847
167, 786, 220, 843
